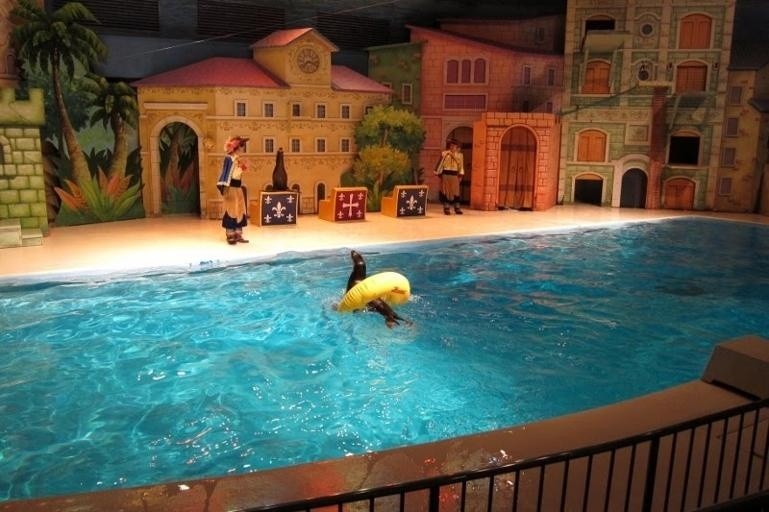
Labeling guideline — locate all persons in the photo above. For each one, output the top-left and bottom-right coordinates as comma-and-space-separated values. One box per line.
216, 136, 251, 244
432, 138, 465, 215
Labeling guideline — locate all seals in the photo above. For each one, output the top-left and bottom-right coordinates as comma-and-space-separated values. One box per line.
346, 249, 412, 327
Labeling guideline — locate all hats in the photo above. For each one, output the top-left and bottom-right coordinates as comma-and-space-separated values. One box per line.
447, 138, 459, 145
226, 137, 249, 155
